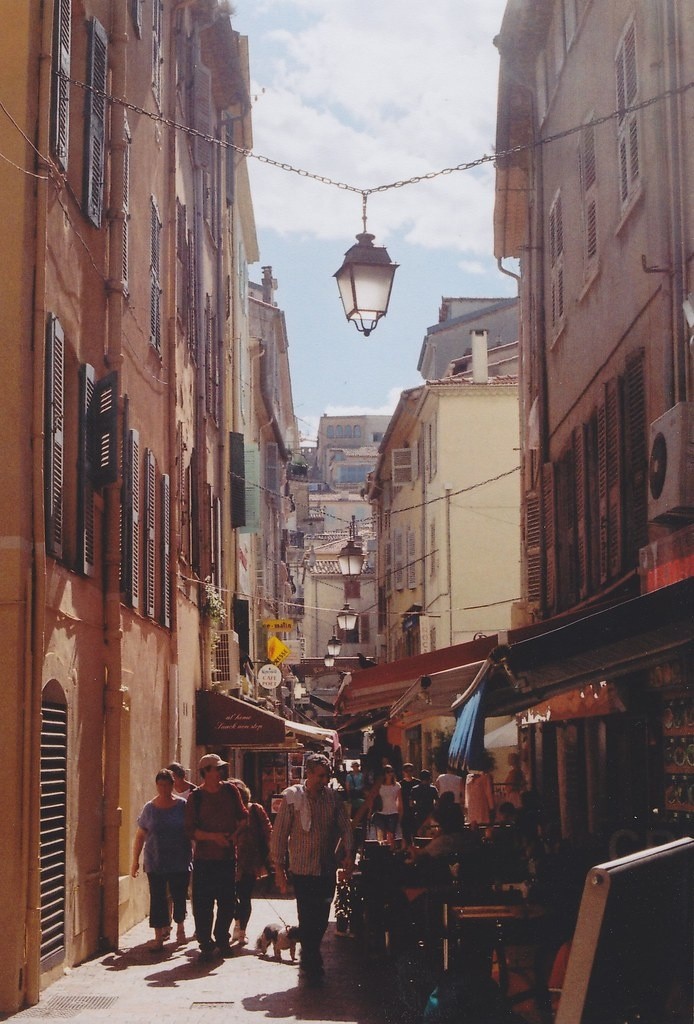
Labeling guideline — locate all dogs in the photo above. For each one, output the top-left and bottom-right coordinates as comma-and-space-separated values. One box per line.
255, 923, 301, 963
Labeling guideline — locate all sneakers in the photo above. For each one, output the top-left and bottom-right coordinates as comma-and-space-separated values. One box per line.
232, 930, 248, 945
219, 947, 234, 958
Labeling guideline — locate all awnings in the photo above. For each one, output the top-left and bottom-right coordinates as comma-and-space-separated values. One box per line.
383, 653, 493, 728
441, 576, 694, 770
328, 591, 635, 715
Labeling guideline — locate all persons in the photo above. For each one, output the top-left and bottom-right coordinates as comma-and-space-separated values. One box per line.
132, 766, 188, 957
227, 777, 273, 947
273, 753, 354, 980
185, 754, 249, 960
162, 760, 199, 941
343, 752, 558, 918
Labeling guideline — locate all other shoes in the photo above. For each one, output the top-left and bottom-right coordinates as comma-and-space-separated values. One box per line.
161, 931, 170, 941
150, 940, 165, 951
177, 932, 188, 944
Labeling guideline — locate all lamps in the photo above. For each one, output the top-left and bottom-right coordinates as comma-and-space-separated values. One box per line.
331, 195, 403, 336
336, 526, 365, 582
336, 590, 358, 630
327, 625, 342, 656
324, 653, 334, 667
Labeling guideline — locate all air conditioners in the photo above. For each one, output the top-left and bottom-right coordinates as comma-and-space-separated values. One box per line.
647, 402, 694, 528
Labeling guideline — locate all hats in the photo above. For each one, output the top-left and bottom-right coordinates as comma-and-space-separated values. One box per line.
197, 753, 230, 771
